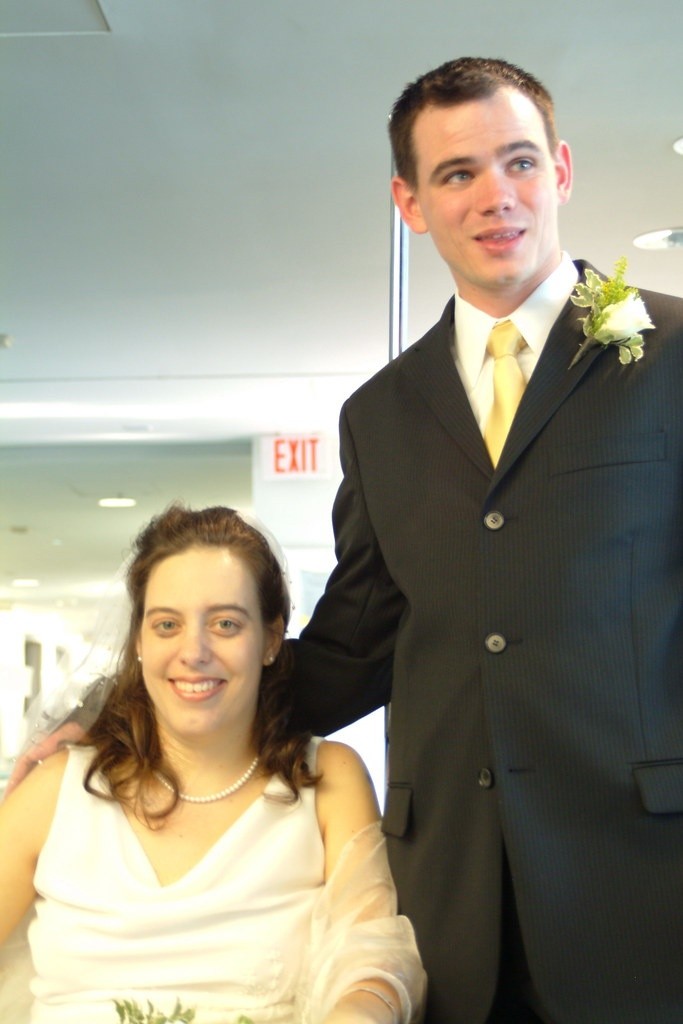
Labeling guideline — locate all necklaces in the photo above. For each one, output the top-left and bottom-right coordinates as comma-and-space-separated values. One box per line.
152, 758, 258, 802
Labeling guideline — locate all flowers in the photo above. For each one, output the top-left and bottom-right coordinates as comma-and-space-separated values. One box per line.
568, 258, 656, 373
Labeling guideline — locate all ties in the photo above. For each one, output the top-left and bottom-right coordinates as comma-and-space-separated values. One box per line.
482, 320, 529, 469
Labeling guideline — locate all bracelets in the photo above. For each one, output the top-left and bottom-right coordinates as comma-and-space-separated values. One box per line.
336, 987, 401, 1024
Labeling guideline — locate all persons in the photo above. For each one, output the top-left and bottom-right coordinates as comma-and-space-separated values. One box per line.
0, 505, 429, 1024
7, 57, 683, 1024
